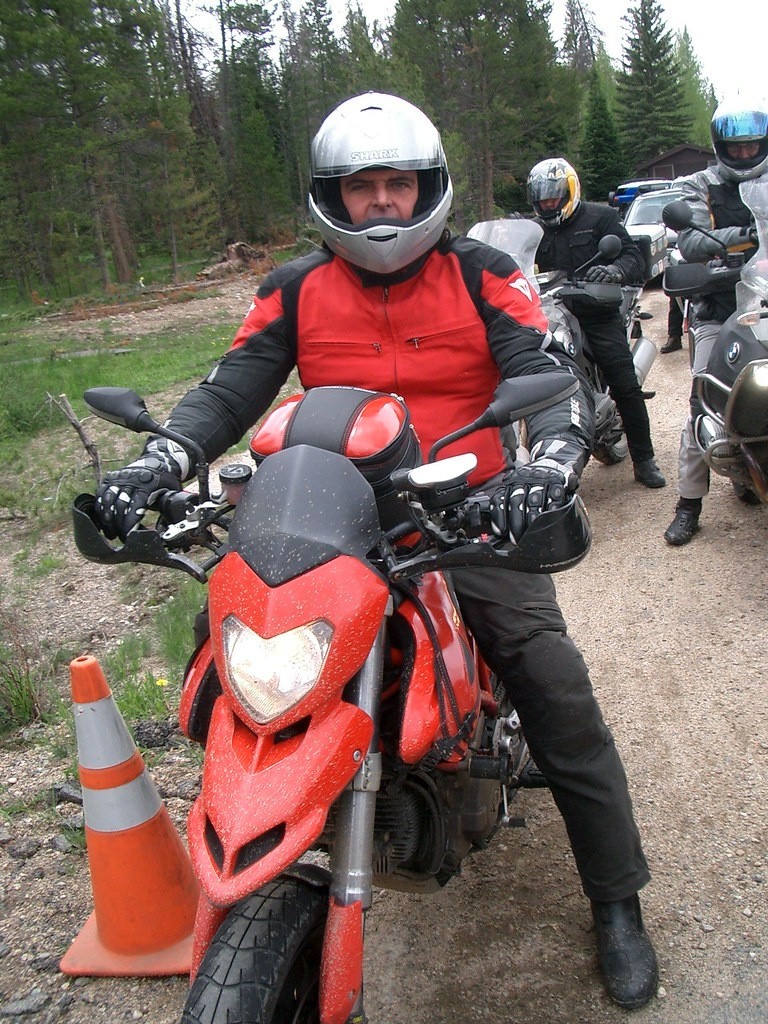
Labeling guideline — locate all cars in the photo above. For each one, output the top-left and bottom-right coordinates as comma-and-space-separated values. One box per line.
608, 177, 679, 285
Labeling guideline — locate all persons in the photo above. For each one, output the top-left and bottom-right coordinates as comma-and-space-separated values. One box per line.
660, 298, 685, 353
497, 159, 665, 488
96, 90, 659, 1006
662, 97, 768, 546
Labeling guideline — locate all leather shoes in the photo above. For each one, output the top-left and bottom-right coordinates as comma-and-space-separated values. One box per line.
634, 460, 665, 488
664, 496, 701, 545
592, 898, 658, 1009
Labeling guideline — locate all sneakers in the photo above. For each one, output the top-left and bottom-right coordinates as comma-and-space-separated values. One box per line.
660, 334, 682, 354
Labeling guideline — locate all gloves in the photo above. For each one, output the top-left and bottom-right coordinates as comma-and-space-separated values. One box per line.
94, 438, 191, 544
489, 437, 586, 545
587, 264, 626, 283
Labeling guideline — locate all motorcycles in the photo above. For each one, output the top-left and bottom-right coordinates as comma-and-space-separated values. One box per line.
463, 217, 661, 467
64, 357, 595, 1023
656, 175, 768, 529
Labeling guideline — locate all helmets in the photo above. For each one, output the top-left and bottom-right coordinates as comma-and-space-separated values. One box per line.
309, 91, 453, 274
711, 98, 768, 182
526, 158, 581, 228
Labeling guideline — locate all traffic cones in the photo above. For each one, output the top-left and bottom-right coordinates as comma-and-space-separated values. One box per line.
55, 654, 197, 980
